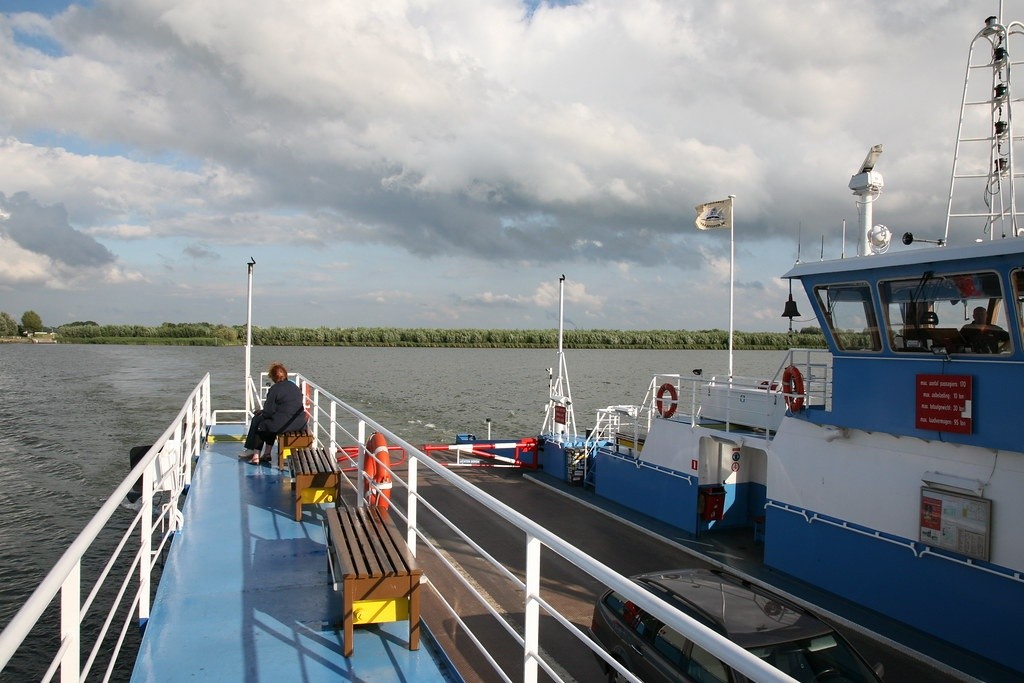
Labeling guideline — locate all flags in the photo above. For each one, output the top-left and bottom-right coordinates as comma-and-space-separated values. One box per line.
695, 200, 732, 231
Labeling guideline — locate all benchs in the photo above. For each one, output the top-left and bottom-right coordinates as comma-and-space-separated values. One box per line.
278, 428, 313, 472
321, 505, 424, 657
289, 445, 342, 522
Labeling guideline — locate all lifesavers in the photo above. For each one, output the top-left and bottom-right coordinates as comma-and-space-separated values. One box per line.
781, 364, 804, 413
656, 383, 677, 418
363, 432, 392, 510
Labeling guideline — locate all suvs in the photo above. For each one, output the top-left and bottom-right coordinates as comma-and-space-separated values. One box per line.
586, 567, 888, 683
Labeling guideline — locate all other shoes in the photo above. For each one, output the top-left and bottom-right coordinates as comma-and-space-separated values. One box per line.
238, 449, 254, 457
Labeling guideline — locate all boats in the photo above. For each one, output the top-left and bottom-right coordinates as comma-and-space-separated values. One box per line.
595, 1, 1024, 683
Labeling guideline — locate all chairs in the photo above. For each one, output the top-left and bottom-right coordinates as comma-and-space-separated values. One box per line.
770, 642, 818, 683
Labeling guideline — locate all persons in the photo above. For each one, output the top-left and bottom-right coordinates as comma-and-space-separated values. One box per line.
238, 364, 308, 465
962, 307, 1011, 354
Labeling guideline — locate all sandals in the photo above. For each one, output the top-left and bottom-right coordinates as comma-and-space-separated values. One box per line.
260, 453, 271, 461
248, 457, 259, 465
